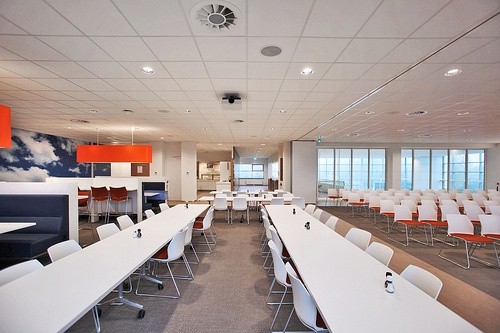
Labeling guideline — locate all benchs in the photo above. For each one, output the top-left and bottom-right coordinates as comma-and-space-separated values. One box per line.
142, 182, 166, 207
0, 194, 69, 268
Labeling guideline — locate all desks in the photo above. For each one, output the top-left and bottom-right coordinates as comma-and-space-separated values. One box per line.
0, 223, 36, 235
265, 205, 484, 333
143, 193, 160, 204
197, 196, 292, 223
1, 203, 211, 333
208, 191, 277, 197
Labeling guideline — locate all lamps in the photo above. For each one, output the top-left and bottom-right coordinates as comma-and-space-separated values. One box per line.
77, 129, 152, 164
0, 104, 12, 148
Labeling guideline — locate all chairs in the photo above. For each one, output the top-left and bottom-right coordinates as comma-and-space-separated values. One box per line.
0, 186, 500, 333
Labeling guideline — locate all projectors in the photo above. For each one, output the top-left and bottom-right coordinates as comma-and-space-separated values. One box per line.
221, 96, 242, 111
